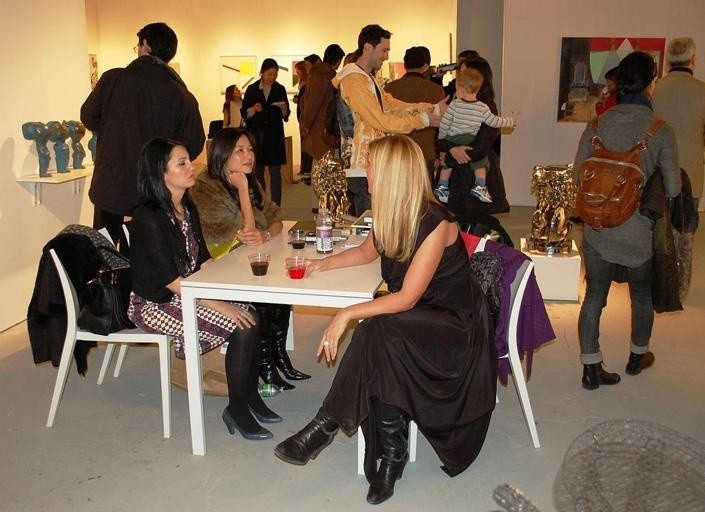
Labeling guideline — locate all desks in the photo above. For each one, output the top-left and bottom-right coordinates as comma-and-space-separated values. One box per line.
180, 221, 384, 475
205, 135, 293, 184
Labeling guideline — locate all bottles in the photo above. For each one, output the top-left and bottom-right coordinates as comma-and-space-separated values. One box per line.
258, 383, 284, 398
315, 208, 335, 254
437, 62, 458, 73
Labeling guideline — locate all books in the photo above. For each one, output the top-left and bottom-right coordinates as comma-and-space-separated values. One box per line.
351, 210, 373, 236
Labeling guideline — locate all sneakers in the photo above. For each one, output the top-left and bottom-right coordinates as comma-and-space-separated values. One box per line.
471, 185, 493, 202
434, 185, 449, 203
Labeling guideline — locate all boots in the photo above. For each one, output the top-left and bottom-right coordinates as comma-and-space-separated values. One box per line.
273, 406, 341, 467
582, 362, 621, 389
366, 412, 410, 505
255, 338, 296, 390
625, 350, 654, 376
272, 328, 312, 381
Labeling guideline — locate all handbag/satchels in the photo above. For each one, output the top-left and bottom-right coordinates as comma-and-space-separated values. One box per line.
77, 269, 137, 337
471, 249, 503, 313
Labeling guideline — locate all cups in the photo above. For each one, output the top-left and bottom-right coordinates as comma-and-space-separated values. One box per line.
288, 230, 306, 249
246, 253, 271, 276
286, 254, 307, 280
545, 244, 556, 255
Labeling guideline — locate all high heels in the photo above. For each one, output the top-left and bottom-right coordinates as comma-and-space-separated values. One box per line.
222, 406, 273, 440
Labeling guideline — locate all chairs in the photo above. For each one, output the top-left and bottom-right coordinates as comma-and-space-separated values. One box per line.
355, 237, 541, 474
46, 227, 183, 439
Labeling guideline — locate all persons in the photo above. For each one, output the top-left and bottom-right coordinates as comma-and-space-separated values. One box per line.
189, 127, 310, 389
571, 51, 682, 391
127, 138, 282, 440
292, 44, 346, 185
648, 38, 704, 307
331, 25, 450, 217
80, 21, 205, 244
595, 67, 618, 116
223, 84, 246, 127
432, 50, 516, 224
383, 45, 446, 188
240, 58, 291, 208
275, 135, 498, 505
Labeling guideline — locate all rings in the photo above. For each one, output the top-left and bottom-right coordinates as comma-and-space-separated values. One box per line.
322, 341, 329, 347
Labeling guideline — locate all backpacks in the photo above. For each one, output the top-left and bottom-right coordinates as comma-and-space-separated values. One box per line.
575, 115, 667, 231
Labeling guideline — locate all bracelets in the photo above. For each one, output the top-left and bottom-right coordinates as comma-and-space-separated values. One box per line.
264, 228, 272, 241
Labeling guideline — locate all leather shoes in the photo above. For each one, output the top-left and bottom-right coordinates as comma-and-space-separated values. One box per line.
249, 403, 283, 423
